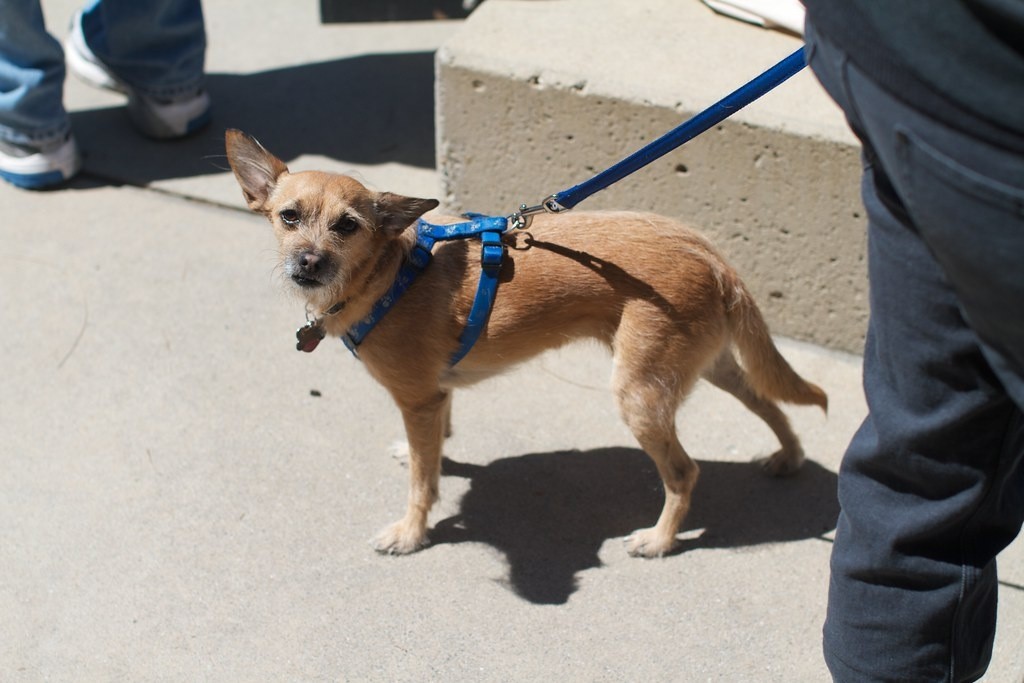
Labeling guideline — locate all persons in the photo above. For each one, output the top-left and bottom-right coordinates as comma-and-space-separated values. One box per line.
799, 1, 1024, 683
0, 0, 212, 190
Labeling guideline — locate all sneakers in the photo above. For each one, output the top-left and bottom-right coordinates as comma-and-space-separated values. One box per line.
65, 9, 212, 140
0, 129, 85, 190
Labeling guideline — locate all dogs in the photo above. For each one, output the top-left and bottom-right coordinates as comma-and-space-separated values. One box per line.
223, 126, 829, 559
